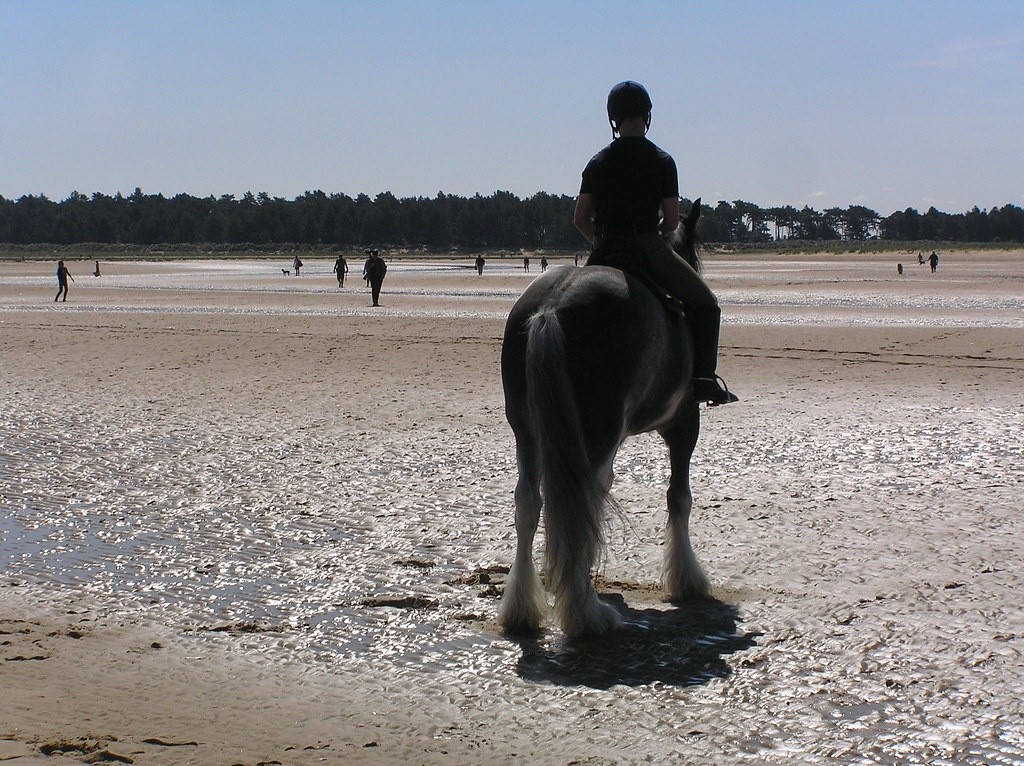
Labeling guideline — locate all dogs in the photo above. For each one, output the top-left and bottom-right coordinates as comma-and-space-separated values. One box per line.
93, 271, 101, 277
282, 269, 290, 275
898, 264, 903, 274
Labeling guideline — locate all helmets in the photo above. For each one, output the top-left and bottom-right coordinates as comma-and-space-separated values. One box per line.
608, 81, 653, 117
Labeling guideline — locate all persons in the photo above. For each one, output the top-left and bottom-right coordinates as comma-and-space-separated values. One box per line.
523, 257, 529, 273
917, 252, 922, 264
95, 261, 101, 277
927, 250, 938, 273
293, 256, 301, 276
574, 81, 740, 404
476, 254, 485, 276
334, 254, 348, 288
364, 248, 386, 306
540, 256, 548, 272
574, 255, 578, 266
55, 261, 75, 302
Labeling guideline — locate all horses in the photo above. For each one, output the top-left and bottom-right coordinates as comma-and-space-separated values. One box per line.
496, 197, 712, 634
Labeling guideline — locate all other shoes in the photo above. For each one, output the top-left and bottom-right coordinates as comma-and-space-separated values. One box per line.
694, 378, 739, 405
